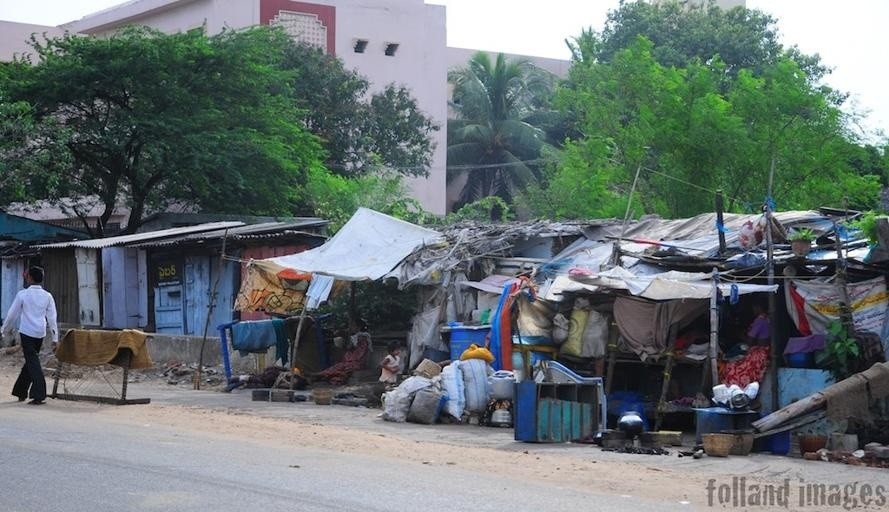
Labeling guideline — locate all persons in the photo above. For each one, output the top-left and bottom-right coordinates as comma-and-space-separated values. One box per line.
720, 298, 769, 388
379, 340, 402, 394
227, 366, 301, 388
0, 266, 60, 404
680, 286, 725, 346
304, 318, 373, 387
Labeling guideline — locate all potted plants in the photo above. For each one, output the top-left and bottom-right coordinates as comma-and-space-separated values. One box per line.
786, 227, 819, 259
